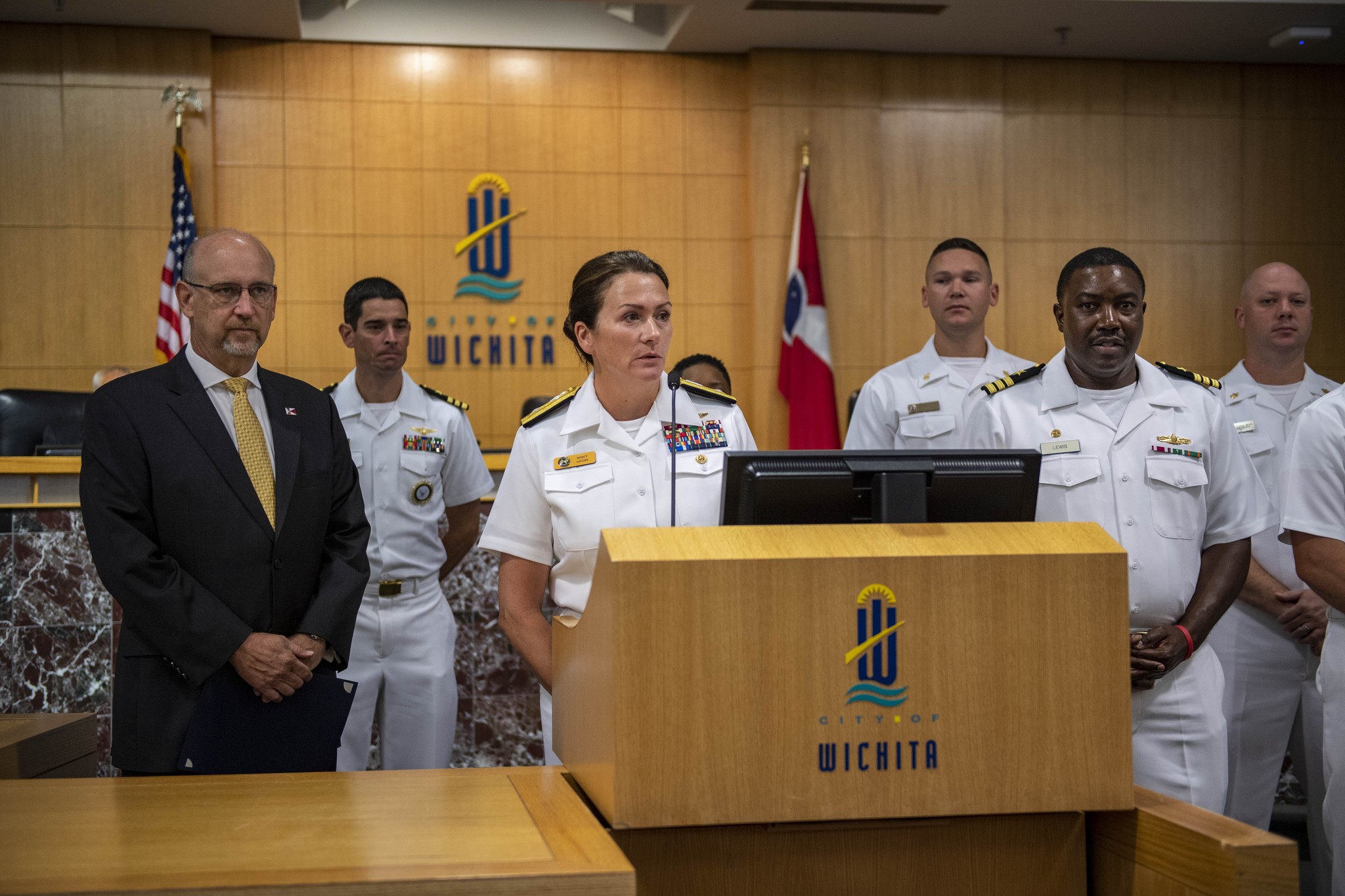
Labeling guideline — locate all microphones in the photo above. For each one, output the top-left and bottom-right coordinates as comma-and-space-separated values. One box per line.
667, 371, 681, 526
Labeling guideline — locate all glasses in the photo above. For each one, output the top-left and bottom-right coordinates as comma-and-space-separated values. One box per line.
186, 280, 277, 305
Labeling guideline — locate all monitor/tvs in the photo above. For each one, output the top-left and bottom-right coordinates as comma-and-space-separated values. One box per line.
717, 449, 1042, 526
0, 387, 89, 457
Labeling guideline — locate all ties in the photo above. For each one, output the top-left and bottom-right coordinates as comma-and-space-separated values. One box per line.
222, 378, 276, 534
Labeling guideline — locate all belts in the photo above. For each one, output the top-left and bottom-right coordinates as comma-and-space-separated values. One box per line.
363, 570, 441, 597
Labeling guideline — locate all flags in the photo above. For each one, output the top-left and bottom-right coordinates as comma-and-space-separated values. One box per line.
777, 173, 845, 449
154, 144, 197, 362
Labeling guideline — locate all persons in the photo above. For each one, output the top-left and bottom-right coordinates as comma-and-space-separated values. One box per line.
843, 238, 1041, 449
960, 247, 1279, 817
1275, 380, 1345, 896
93, 367, 134, 394
672, 353, 732, 396
477, 249, 757, 767
313, 273, 495, 771
78, 229, 372, 776
1206, 262, 1343, 896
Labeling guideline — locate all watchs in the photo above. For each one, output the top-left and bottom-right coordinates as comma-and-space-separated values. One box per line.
311, 634, 330, 650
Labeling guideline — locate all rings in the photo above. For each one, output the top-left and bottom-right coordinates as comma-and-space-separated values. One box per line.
1301, 624, 1311, 633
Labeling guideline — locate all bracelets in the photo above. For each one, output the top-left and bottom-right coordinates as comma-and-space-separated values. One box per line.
1176, 624, 1193, 661
